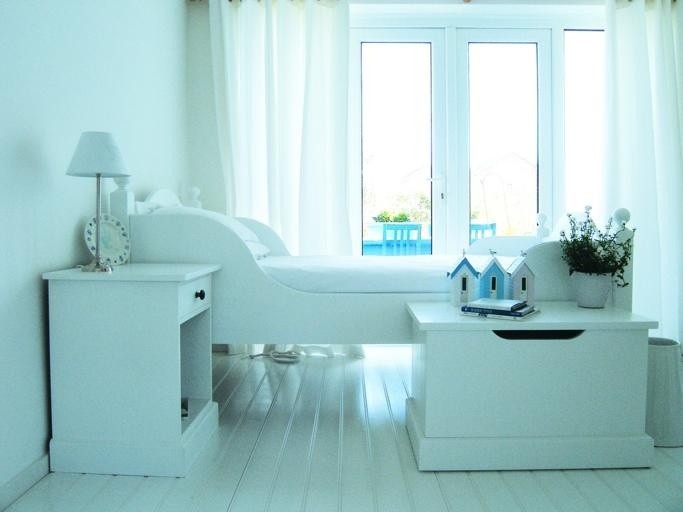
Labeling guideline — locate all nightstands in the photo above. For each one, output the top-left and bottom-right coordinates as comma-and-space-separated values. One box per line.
41, 262, 222, 477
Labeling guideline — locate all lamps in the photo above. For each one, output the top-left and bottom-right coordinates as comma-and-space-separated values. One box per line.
66, 132, 132, 273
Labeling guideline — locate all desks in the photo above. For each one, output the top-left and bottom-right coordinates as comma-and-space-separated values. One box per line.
405, 301, 660, 471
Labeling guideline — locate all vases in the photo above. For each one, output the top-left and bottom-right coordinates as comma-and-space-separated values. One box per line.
573, 269, 612, 309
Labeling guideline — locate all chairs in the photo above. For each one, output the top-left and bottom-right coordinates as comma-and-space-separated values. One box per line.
382, 224, 421, 255
470, 223, 496, 246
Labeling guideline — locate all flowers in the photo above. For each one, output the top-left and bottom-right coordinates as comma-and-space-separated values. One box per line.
556, 204, 637, 288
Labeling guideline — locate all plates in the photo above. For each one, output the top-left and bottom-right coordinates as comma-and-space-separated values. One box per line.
84, 214, 131, 266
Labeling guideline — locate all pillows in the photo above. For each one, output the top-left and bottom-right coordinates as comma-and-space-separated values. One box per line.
149, 195, 270, 258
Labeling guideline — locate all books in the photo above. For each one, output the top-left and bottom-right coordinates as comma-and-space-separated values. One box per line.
462, 308, 539, 321
460, 304, 533, 316
465, 297, 527, 311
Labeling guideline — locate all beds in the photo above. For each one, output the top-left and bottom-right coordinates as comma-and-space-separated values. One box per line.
111, 177, 634, 344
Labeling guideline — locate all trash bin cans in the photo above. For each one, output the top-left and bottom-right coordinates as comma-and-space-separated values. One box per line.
646, 337, 683, 448
